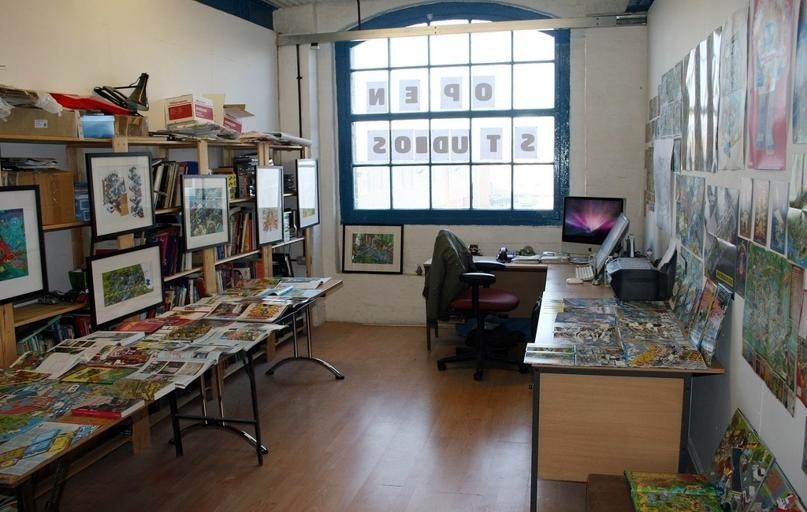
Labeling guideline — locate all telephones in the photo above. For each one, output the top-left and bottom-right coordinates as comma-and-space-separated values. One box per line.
496, 247, 514, 263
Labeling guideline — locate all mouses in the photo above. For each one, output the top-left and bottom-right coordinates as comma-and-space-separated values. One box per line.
566, 278, 583, 285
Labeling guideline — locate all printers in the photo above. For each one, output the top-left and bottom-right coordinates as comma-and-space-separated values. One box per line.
605, 245, 677, 301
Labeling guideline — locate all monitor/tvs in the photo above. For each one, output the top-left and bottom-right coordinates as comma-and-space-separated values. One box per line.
562, 197, 626, 263
588, 212, 628, 275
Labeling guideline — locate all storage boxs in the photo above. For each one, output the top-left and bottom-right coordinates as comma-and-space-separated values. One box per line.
9, 170, 77, 225
80, 115, 115, 140
201, 93, 256, 135
0, 107, 79, 137
164, 94, 215, 127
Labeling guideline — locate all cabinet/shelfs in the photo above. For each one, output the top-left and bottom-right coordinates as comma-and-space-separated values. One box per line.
0, 134, 312, 512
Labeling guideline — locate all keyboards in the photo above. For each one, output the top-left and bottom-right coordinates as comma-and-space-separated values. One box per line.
576, 266, 593, 281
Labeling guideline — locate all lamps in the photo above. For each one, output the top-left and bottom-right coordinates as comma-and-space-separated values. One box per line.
93, 73, 149, 118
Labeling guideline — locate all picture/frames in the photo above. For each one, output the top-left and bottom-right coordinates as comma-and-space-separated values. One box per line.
86, 151, 155, 243
179, 174, 231, 252
342, 224, 404, 274
0, 184, 49, 306
296, 159, 320, 229
254, 165, 285, 248
87, 242, 166, 331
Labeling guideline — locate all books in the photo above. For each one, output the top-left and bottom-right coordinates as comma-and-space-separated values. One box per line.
16, 310, 93, 358
0, 396, 65, 442
216, 204, 259, 258
128, 343, 233, 393
523, 298, 707, 369
70, 394, 145, 419
151, 159, 200, 210
62, 366, 140, 385
667, 265, 732, 367
745, 459, 807, 512
235, 154, 275, 199
711, 409, 775, 512
1, 421, 102, 476
91, 376, 175, 402
1, 158, 75, 226
76, 330, 145, 360
624, 471, 724, 512
97, 222, 203, 324
11, 338, 113, 381
100, 340, 190, 368
120, 277, 331, 351
0, 368, 52, 394
10, 381, 88, 415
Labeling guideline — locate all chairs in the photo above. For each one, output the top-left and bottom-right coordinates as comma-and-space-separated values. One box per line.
435, 230, 529, 382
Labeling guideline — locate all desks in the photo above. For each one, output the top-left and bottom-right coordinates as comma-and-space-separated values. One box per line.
423, 255, 548, 352
0, 280, 344, 512
531, 263, 727, 512
